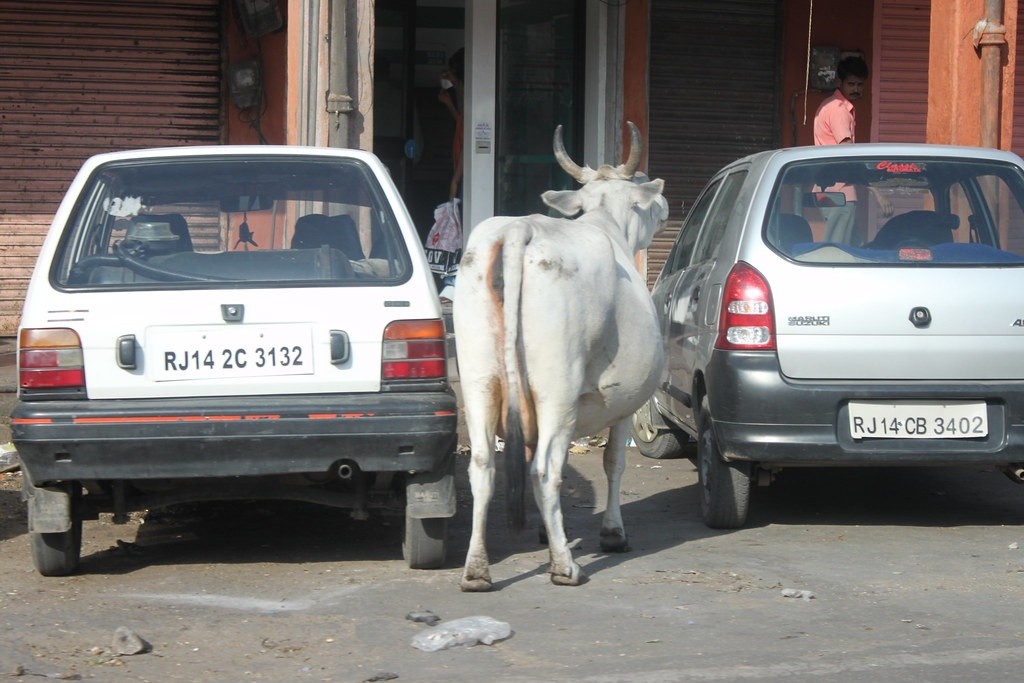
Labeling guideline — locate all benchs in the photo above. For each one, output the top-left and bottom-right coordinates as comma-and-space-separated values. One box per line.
84, 247, 355, 285
786, 242, 1024, 265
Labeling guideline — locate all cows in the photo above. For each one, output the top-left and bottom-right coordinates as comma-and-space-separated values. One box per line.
452, 119, 670, 593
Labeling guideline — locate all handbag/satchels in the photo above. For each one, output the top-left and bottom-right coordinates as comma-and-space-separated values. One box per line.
423, 198, 463, 276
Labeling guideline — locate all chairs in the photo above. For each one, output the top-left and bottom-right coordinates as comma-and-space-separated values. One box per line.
123, 214, 192, 252
770, 213, 814, 253
291, 214, 365, 260
861, 210, 954, 249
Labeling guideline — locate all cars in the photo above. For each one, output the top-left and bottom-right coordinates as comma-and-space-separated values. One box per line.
627, 142, 1024, 530
6, 143, 459, 577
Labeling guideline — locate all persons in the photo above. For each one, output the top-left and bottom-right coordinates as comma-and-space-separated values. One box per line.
812, 56, 893, 244
448, 47, 465, 201
374, 58, 424, 200
499, 66, 551, 215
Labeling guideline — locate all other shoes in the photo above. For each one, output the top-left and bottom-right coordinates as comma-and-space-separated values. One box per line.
439, 285, 454, 302
